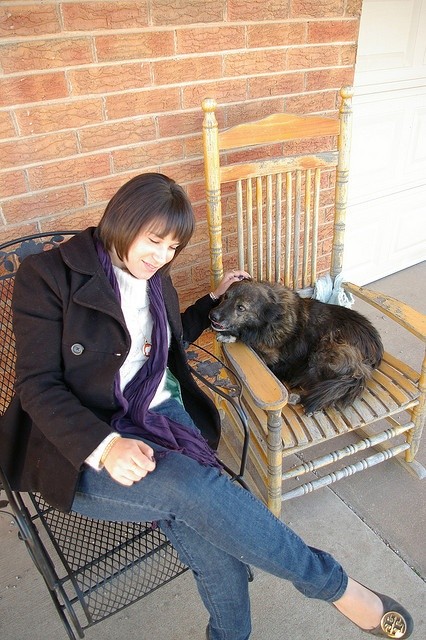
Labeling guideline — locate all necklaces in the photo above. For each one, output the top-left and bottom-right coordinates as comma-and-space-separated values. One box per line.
140, 329, 152, 356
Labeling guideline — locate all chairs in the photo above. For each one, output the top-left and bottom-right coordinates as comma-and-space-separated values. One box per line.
0, 229, 253, 639
203, 86, 426, 519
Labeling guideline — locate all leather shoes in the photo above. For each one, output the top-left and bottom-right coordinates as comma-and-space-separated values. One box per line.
328, 576, 414, 639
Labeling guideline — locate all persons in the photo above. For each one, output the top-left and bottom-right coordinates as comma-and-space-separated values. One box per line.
0, 172, 415, 640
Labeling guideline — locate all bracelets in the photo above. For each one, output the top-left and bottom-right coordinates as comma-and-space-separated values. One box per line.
209, 291, 216, 301
100, 436, 121, 463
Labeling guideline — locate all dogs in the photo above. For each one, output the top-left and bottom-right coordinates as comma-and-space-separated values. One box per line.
209, 278, 384, 417
